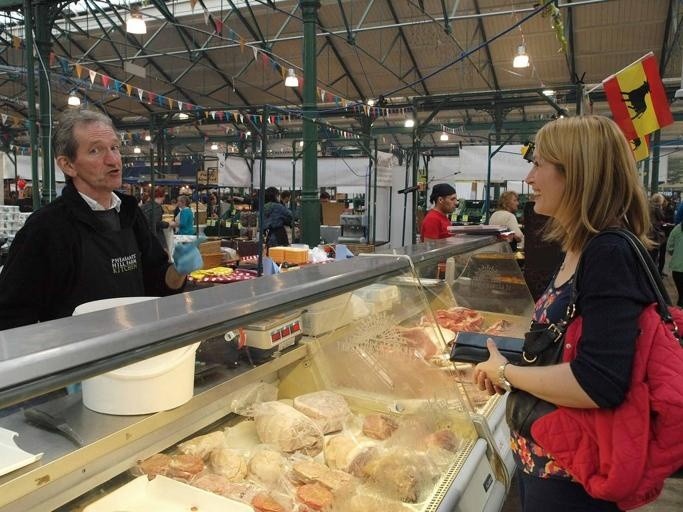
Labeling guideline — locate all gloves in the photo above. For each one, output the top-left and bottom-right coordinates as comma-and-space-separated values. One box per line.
172, 236, 206, 276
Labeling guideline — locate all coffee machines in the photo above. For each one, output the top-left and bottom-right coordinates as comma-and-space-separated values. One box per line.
336, 212, 368, 244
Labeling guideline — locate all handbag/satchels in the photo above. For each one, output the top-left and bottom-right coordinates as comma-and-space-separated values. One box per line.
505, 226, 683, 479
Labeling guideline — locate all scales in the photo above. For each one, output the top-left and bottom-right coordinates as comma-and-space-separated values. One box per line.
242, 309, 304, 359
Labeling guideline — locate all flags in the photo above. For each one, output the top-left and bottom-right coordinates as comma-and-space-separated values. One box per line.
629, 134, 652, 163
601, 50, 675, 140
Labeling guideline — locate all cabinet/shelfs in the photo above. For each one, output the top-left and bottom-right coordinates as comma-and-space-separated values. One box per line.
0, 231, 536, 512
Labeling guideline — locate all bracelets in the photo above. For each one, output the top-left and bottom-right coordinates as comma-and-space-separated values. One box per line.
497, 361, 512, 393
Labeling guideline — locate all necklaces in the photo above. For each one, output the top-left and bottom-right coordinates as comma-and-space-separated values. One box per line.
559, 246, 579, 272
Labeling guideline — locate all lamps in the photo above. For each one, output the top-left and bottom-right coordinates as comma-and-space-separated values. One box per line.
124, 0, 146, 36
512, 46, 530, 69
439, 131, 448, 141
284, 68, 299, 89
67, 90, 81, 107
403, 117, 414, 127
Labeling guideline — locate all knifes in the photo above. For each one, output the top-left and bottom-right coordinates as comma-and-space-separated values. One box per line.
17, 403, 89, 449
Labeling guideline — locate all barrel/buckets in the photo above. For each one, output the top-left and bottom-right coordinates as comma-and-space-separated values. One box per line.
74, 294, 203, 417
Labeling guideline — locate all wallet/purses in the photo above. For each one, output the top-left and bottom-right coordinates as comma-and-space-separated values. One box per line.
450, 331, 525, 366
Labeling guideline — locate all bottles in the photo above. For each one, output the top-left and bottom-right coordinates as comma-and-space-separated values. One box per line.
437, 257, 446, 280
344, 193, 365, 215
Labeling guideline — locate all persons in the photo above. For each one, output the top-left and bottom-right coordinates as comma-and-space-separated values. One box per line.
0, 104, 210, 331
120, 187, 334, 253
650, 191, 682, 308
471, 113, 673, 511
418, 181, 458, 243
487, 190, 524, 251
4, 185, 33, 212
